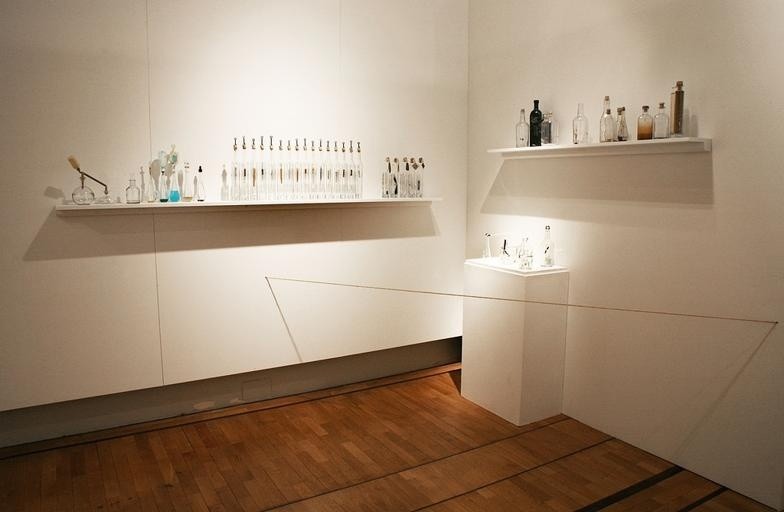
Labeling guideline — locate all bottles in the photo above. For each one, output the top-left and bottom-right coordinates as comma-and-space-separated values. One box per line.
228, 134, 362, 199
482, 225, 554, 272
513, 83, 687, 146
382, 157, 423, 197
73, 163, 207, 203
